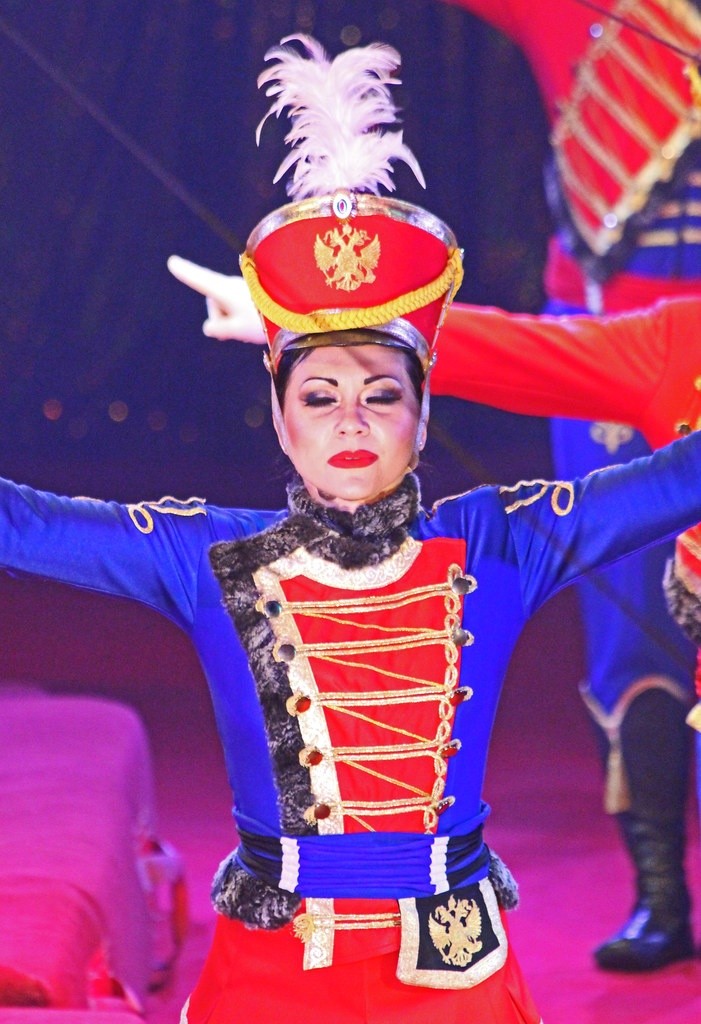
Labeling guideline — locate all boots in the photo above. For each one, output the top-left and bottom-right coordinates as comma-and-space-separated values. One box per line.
576, 678, 699, 975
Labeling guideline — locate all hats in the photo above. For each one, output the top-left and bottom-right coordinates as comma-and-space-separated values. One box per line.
240, 36, 466, 377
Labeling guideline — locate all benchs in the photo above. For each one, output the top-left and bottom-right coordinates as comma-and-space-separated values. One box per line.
1, 696, 184, 1022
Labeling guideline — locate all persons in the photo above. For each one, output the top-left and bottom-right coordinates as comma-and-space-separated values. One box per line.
166, 253, 701, 803
458, 0, 701, 970
0, 192, 701, 1024
